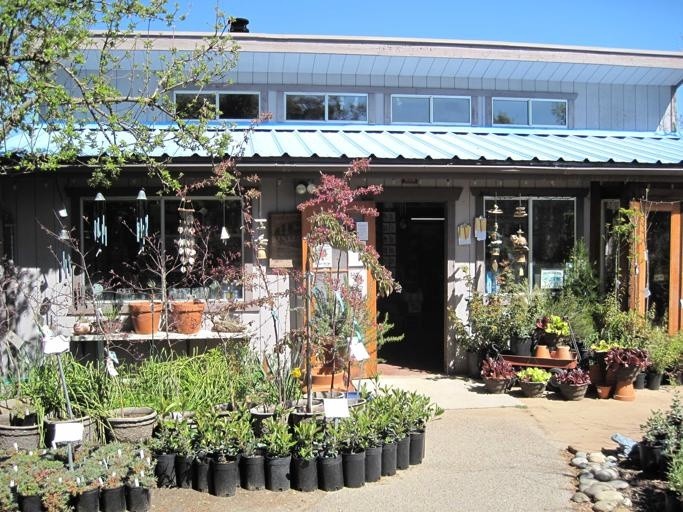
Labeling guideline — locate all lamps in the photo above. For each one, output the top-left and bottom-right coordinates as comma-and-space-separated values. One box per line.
293, 178, 318, 195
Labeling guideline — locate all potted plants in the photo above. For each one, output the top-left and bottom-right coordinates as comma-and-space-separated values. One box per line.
154, 361, 445, 496
481, 311, 665, 400
0, 361, 445, 512
0, 361, 153, 512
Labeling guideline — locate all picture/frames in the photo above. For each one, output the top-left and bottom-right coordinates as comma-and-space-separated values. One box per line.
265, 211, 306, 276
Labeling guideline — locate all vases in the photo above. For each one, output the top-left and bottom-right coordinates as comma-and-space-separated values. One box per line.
127, 299, 162, 337
167, 298, 205, 332
